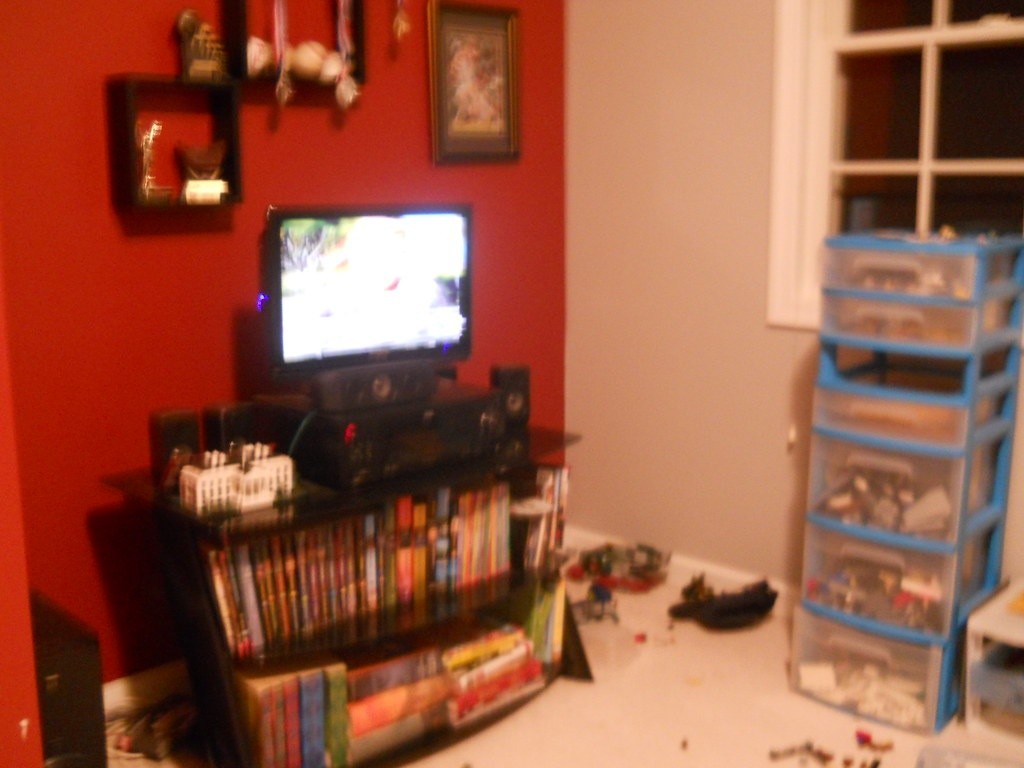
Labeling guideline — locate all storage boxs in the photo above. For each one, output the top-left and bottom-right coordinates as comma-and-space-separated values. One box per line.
963, 579, 1024, 750
821, 245, 1015, 299
821, 295, 1013, 349
808, 434, 992, 542
788, 606, 942, 738
812, 385, 1002, 446
801, 523, 987, 635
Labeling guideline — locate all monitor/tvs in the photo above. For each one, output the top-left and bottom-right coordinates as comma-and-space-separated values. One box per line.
260, 202, 475, 382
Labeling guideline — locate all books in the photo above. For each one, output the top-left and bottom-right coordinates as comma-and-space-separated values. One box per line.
207, 466, 571, 657
234, 620, 546, 768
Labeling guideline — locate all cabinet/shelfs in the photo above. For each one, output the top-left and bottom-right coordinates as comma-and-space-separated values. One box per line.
800, 227, 1022, 732
101, 425, 593, 768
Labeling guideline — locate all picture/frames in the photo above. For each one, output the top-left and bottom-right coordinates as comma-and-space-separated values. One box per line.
425, 0, 522, 164
